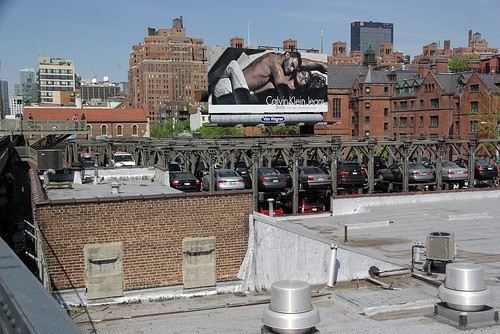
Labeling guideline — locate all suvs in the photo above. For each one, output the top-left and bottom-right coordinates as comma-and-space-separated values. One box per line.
321, 159, 365, 187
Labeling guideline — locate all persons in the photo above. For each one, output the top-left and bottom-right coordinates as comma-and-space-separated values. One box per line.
241, 50, 327, 97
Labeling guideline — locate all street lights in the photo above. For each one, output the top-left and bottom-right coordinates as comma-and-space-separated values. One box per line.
17, 99, 23, 133
82, 101, 87, 131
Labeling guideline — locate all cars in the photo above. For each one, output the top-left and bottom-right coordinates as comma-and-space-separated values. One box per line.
350, 155, 388, 176
169, 172, 201, 192
241, 167, 287, 192
168, 162, 185, 172
280, 165, 333, 189
200, 168, 245, 191
258, 183, 418, 215
452, 158, 499, 179
376, 161, 434, 183
111, 152, 136, 168
426, 160, 469, 181
